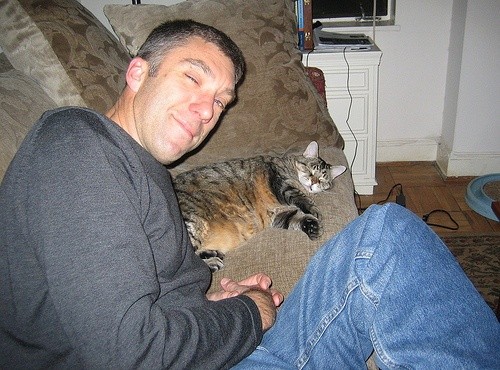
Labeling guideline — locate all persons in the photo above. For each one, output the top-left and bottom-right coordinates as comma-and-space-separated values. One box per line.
0, 20, 500, 370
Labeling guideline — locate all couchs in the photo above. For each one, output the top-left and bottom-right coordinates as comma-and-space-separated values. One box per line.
0, 0, 380, 370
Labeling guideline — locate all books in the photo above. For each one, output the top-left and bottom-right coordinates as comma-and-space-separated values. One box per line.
294, 0, 313, 50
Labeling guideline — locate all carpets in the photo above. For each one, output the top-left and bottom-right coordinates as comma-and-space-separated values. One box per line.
442, 237, 500, 322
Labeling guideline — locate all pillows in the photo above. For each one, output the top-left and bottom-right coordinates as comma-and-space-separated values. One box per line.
103, 0, 346, 173
0, 0, 130, 112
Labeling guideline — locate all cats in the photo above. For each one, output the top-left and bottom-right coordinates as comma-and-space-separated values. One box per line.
172, 140, 346, 273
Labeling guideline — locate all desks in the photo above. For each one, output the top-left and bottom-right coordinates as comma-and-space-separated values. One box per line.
299, 38, 383, 196
